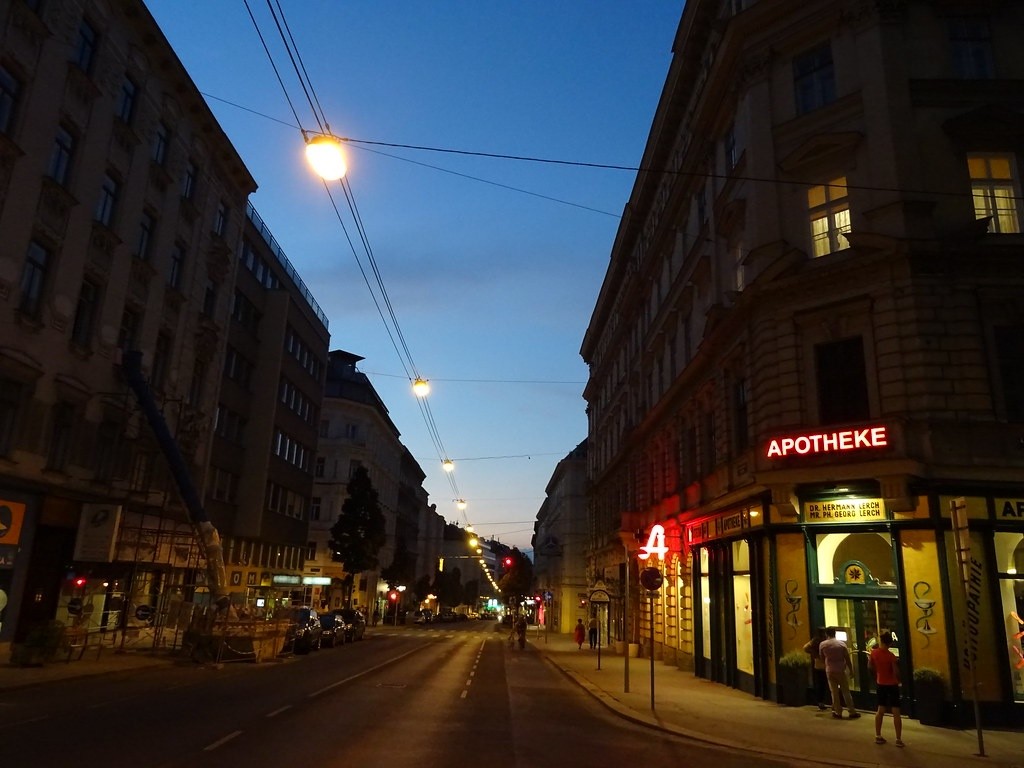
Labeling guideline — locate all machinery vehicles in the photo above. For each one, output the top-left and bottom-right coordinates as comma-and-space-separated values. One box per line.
112, 349, 290, 664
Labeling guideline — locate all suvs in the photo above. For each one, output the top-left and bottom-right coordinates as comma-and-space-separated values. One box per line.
273, 605, 322, 654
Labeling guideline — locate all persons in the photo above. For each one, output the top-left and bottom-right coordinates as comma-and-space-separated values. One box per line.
575, 613, 601, 650
364, 608, 380, 627
868, 631, 906, 748
511, 616, 527, 649
802, 626, 861, 719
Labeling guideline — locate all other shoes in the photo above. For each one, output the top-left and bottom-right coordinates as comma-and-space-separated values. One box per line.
849, 713, 861, 718
833, 712, 842, 719
819, 705, 827, 710
876, 735, 887, 744
896, 740, 905, 747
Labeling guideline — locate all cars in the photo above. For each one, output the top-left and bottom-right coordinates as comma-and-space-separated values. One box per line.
439, 611, 468, 622
422, 609, 432, 620
413, 611, 425, 622
329, 609, 365, 643
318, 614, 345, 648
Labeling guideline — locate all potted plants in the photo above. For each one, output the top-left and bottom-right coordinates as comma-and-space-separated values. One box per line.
913, 668, 947, 727
779, 649, 812, 706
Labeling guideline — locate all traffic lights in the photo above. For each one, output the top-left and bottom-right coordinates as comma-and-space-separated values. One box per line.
388, 590, 399, 601
504, 557, 513, 566
